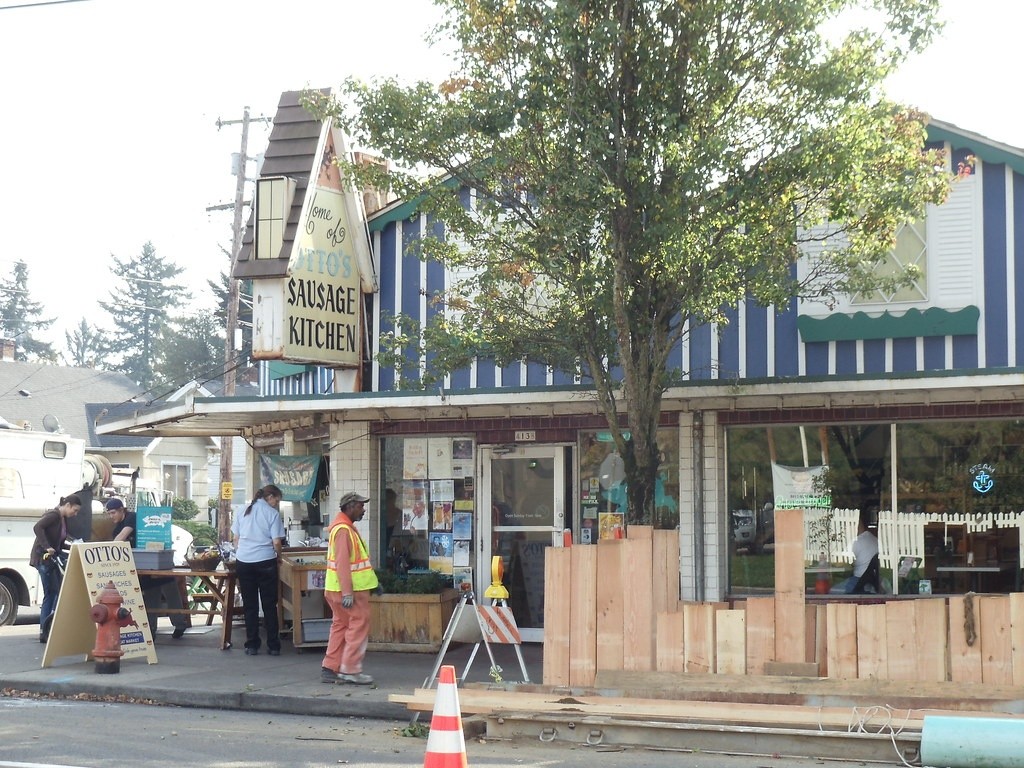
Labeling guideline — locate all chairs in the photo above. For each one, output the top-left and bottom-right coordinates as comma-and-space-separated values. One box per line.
849, 552, 880, 594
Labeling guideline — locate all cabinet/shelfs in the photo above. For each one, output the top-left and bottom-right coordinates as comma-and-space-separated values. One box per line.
759, 431, 1023, 594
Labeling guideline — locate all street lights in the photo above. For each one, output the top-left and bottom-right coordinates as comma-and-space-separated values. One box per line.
18, 389, 61, 431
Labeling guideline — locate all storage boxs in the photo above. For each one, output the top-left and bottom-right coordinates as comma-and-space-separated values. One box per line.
289, 618, 333, 643
128, 549, 176, 570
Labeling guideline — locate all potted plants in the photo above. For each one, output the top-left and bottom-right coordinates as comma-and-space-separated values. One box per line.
368, 567, 461, 654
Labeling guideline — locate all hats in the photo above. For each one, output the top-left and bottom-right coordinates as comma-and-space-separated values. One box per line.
339, 492, 370, 507
106, 499, 123, 511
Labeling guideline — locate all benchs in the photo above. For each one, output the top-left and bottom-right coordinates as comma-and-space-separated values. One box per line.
191, 593, 227, 603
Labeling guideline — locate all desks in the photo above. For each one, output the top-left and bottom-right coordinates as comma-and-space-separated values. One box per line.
937, 560, 1017, 593
137, 569, 245, 650
277, 547, 328, 653
804, 563, 854, 594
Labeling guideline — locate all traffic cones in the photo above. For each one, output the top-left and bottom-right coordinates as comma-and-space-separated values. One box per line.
423, 666, 468, 768
814, 555, 831, 595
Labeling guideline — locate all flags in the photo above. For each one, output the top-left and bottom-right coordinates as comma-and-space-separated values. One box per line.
259, 451, 321, 504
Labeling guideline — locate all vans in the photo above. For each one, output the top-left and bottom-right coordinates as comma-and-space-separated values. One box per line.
492, 501, 514, 576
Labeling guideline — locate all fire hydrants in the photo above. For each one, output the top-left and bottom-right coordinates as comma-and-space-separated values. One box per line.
91, 579, 132, 674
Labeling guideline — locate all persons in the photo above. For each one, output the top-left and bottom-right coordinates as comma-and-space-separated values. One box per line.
454, 442, 467, 458
104, 498, 187, 638
430, 537, 447, 557
409, 500, 429, 531
321, 491, 377, 684
384, 487, 414, 584
230, 484, 286, 657
829, 516, 879, 595
29, 494, 82, 644
456, 514, 467, 525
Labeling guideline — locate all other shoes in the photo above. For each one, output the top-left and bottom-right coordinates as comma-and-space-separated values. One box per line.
38, 635, 48, 643
244, 648, 256, 655
336, 673, 374, 684
172, 621, 187, 638
320, 667, 337, 682
267, 649, 280, 655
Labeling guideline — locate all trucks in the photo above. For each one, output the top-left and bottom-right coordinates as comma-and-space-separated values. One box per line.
0, 418, 194, 626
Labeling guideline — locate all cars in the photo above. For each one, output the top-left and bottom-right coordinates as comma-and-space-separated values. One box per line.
392, 536, 454, 588
733, 503, 774, 552
